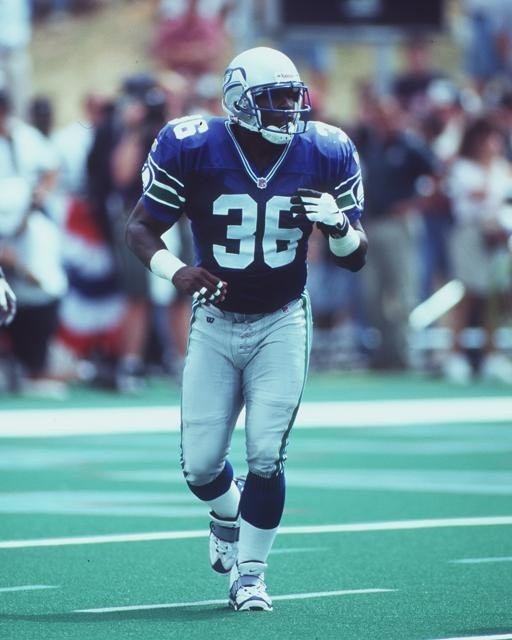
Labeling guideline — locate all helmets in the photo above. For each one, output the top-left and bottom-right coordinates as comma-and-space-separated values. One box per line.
220, 48, 313, 144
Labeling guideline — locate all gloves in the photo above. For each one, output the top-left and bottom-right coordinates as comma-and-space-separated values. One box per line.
290, 188, 348, 239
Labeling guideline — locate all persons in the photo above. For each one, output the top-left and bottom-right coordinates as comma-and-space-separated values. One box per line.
122, 43, 372, 615
2, 1, 512, 405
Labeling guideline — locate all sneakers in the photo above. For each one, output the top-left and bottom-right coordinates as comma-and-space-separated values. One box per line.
227, 559, 273, 611
207, 475, 247, 572
